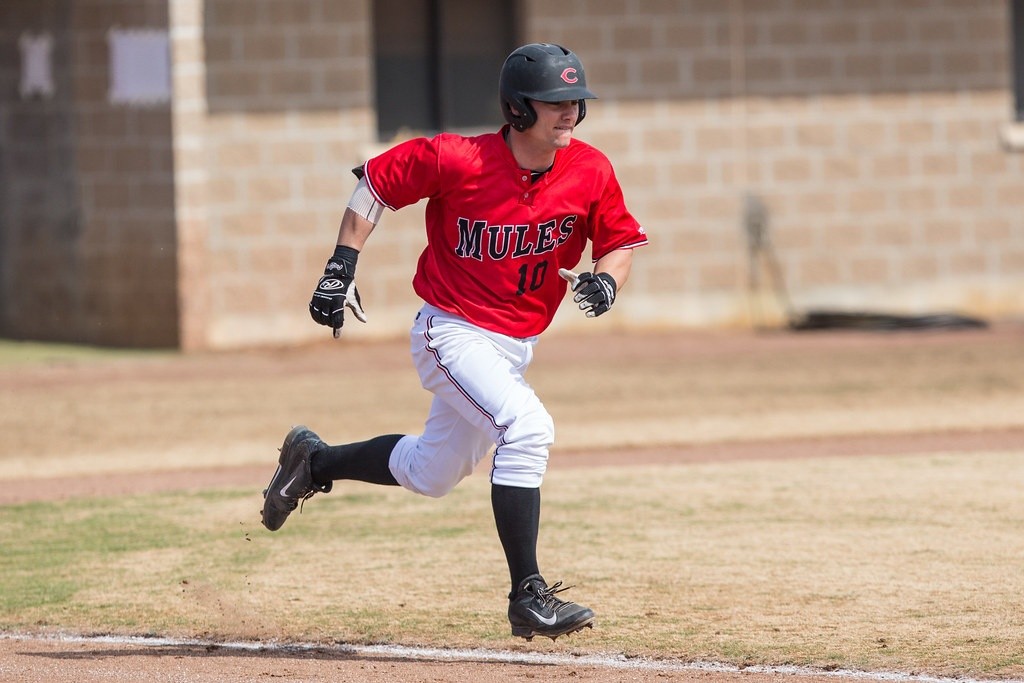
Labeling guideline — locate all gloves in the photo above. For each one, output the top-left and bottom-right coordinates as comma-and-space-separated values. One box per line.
309, 256, 366, 338
558, 268, 616, 317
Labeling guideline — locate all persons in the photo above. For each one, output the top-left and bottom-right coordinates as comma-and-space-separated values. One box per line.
259, 42, 648, 642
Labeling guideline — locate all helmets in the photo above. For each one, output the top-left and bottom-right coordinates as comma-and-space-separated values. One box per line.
500, 42, 598, 133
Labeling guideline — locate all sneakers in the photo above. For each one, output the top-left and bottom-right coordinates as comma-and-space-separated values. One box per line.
508, 574, 595, 641
260, 425, 333, 531
